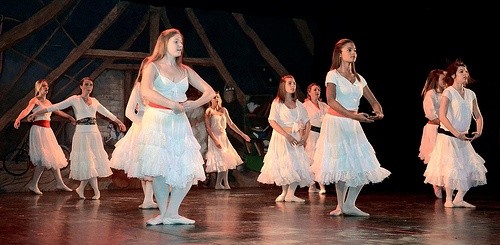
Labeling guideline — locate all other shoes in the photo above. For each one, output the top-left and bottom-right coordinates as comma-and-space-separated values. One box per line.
138, 200, 159, 208
308, 188, 326, 193
28, 185, 43, 195
215, 184, 231, 189
330, 204, 370, 216
432, 184, 443, 199
75, 187, 86, 199
146, 214, 195, 225
56, 184, 73, 192
444, 200, 476, 208
275, 194, 305, 202
92, 190, 100, 199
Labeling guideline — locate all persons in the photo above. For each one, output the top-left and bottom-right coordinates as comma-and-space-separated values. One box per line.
257, 75, 330, 203
112, 28, 217, 227
14, 79, 77, 195
309, 38, 386, 217
205, 91, 252, 190
27, 76, 126, 201
418, 60, 487, 208
